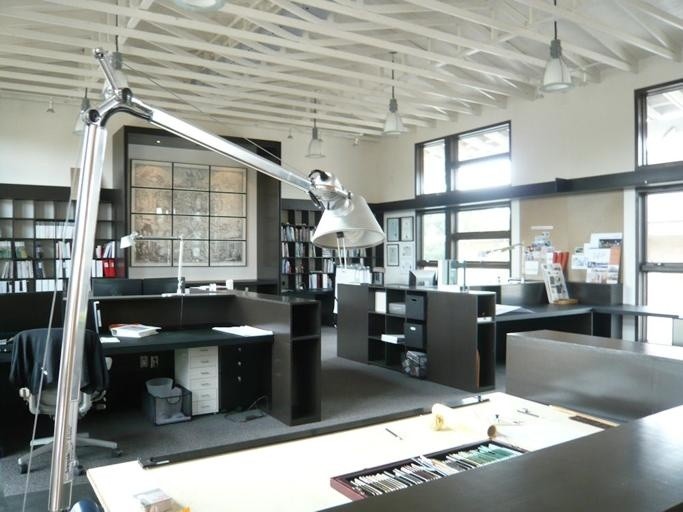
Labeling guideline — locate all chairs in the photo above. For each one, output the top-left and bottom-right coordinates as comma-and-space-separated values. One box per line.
138, 276, 186, 296
91, 278, 142, 296
10, 327, 122, 477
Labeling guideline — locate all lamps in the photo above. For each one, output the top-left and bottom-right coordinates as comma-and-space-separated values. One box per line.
541, 1, 573, 93
305, 93, 328, 161
20, 46, 390, 512
119, 232, 188, 297
381, 51, 409, 136
71, 87, 94, 134
101, 0, 130, 103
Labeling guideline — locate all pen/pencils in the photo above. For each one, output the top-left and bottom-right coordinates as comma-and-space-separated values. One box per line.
517, 409, 539, 417
350, 443, 524, 497
386, 428, 401, 440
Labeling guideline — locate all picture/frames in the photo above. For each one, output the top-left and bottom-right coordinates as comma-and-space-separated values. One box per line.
384, 212, 417, 267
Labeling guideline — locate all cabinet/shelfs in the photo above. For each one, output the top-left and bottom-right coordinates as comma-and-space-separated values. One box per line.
0, 183, 128, 296
173, 345, 219, 416
280, 197, 373, 300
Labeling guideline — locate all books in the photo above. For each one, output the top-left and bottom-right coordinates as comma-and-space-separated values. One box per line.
0, 221, 116, 293
111, 324, 162, 338
281, 226, 383, 289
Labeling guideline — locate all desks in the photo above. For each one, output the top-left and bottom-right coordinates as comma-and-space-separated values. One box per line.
0, 290, 322, 427
336, 265, 682, 408
0, 328, 682, 511
